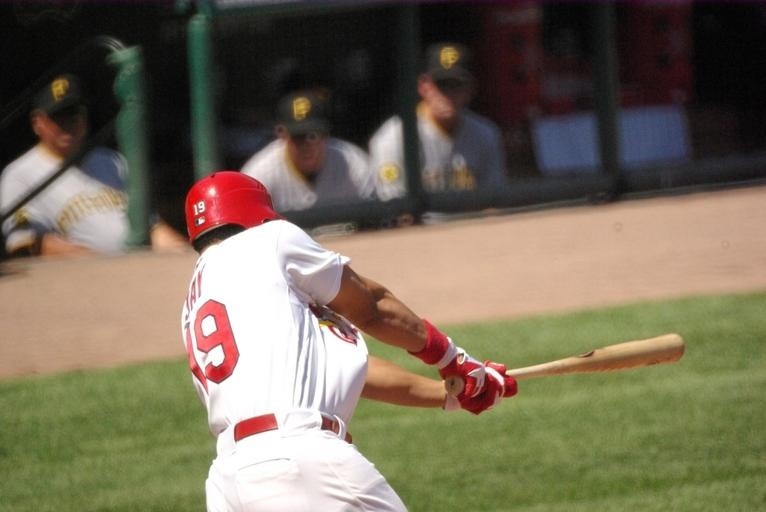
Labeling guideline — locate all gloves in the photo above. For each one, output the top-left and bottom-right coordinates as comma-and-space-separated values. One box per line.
408, 319, 517, 414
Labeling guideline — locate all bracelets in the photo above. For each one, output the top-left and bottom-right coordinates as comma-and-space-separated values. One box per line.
408, 320, 449, 365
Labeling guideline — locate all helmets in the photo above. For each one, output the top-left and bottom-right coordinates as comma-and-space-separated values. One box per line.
185, 171, 285, 249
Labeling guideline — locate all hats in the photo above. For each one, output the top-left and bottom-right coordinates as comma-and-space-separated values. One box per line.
36, 74, 85, 127
424, 45, 472, 83
282, 94, 329, 139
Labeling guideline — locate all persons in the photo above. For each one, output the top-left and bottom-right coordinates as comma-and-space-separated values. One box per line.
368, 42, 508, 226
180, 170, 518, 512
0, 73, 194, 256
240, 90, 376, 215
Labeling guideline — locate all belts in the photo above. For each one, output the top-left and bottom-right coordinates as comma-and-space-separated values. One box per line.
234, 414, 351, 444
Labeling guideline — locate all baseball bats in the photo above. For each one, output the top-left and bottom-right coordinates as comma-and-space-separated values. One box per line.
446, 333, 684, 397
